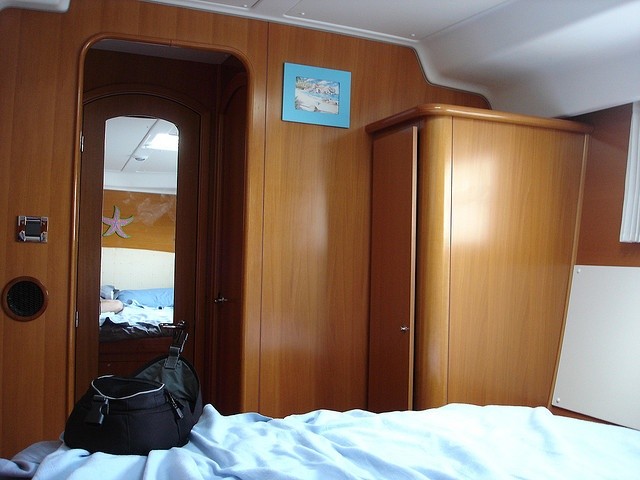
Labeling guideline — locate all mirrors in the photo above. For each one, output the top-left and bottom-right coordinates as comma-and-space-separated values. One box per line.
100, 114, 179, 324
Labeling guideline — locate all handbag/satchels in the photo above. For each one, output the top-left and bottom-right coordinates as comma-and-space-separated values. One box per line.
64, 329, 203, 456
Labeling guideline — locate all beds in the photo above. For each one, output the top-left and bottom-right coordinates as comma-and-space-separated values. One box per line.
1, 265, 640, 479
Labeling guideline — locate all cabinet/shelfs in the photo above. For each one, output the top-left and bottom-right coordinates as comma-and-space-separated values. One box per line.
366, 104, 452, 415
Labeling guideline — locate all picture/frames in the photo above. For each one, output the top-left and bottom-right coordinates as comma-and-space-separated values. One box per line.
279, 62, 351, 129
